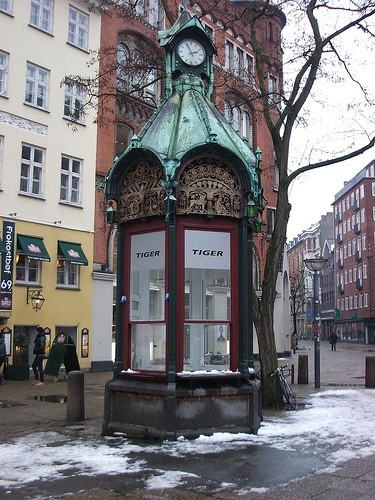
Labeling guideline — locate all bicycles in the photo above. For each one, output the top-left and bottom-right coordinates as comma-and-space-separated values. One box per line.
270, 364, 298, 410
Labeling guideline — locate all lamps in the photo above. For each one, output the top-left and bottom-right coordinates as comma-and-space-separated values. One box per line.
252, 217, 263, 234
27, 287, 45, 312
246, 197, 258, 220
162, 191, 177, 220
105, 202, 117, 230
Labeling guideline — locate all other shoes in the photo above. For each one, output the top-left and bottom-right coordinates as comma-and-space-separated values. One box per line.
35, 381, 44, 385
31, 380, 40, 384
0, 378, 4, 384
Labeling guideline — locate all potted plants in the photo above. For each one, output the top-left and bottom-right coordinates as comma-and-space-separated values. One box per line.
6, 333, 29, 381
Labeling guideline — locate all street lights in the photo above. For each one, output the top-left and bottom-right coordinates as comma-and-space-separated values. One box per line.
301, 253, 329, 389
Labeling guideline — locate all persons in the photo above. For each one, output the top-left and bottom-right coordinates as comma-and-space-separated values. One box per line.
218, 326, 225, 342
32, 327, 46, 386
291, 332, 338, 354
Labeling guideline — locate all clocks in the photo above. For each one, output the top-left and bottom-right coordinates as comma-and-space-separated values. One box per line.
176, 38, 207, 68
